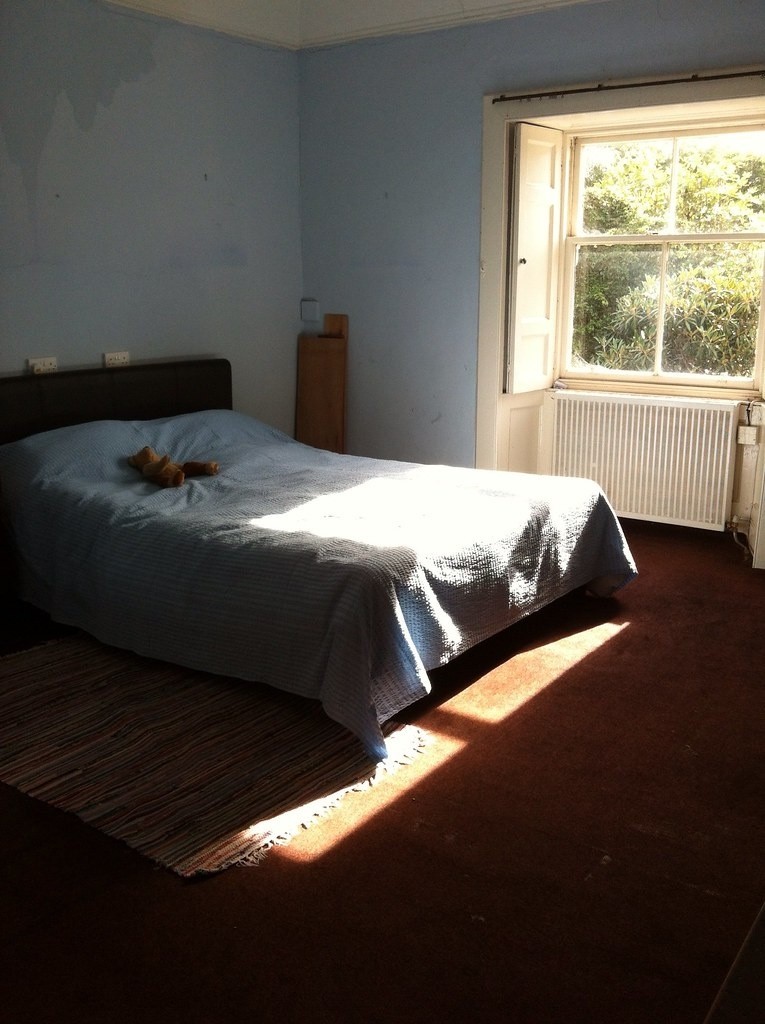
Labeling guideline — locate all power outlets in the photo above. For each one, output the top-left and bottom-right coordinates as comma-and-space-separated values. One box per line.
105, 352, 130, 367
27, 357, 57, 375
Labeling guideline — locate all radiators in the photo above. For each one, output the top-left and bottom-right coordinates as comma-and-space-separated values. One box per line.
547, 388, 741, 532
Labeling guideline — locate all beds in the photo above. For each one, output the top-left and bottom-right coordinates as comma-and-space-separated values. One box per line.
0, 408, 605, 708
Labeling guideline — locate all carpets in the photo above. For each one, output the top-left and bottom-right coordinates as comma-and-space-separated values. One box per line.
2, 636, 424, 878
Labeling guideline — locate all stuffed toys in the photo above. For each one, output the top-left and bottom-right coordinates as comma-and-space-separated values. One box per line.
127, 446, 220, 488
